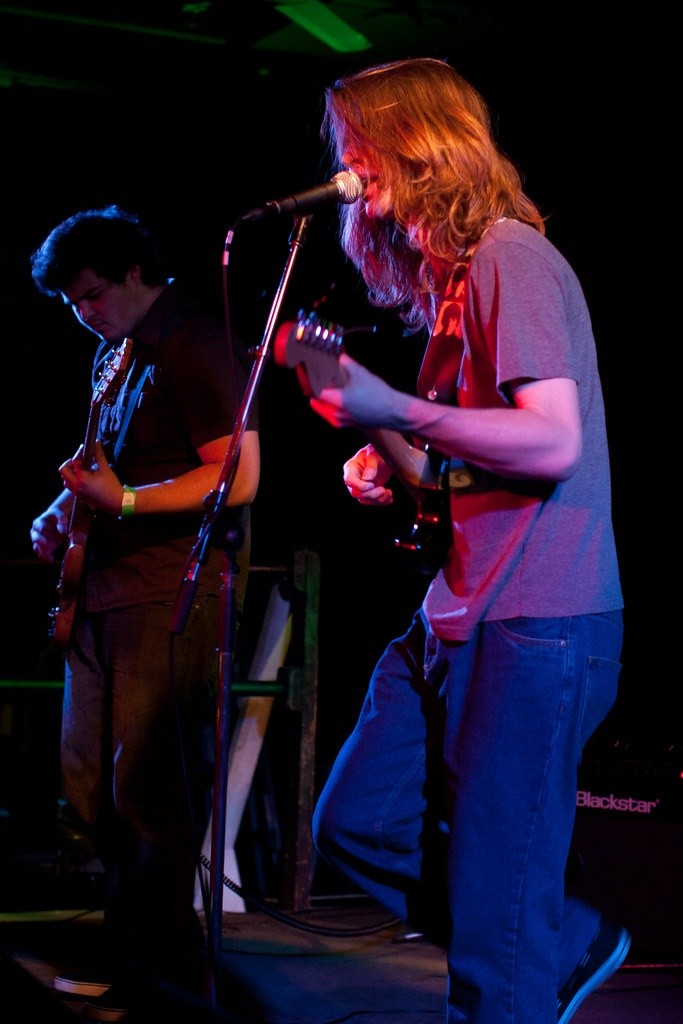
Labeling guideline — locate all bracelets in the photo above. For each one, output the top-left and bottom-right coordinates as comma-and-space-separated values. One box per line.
121, 483, 139, 517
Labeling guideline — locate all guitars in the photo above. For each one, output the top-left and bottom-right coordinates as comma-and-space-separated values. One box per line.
273, 305, 458, 571
44, 335, 137, 646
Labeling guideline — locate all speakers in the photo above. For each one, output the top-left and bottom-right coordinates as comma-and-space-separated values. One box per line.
560, 744, 683, 965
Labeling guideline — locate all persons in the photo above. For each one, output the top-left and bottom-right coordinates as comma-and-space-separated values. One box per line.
309, 58, 634, 1024
26, 203, 261, 1022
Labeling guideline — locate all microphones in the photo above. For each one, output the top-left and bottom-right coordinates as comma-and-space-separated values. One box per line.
243, 170, 364, 220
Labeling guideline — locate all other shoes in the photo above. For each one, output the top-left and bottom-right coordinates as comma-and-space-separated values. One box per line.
87, 967, 152, 1023
558, 917, 632, 1024
52, 968, 112, 998
